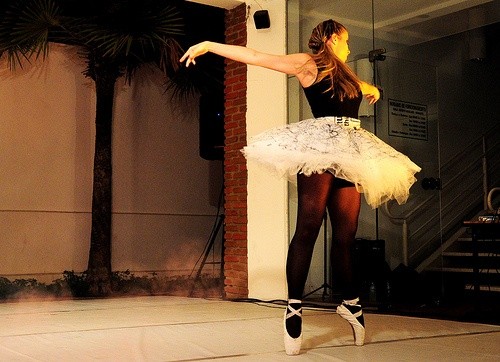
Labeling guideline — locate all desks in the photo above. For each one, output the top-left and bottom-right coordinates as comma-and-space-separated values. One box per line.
463, 219, 500, 297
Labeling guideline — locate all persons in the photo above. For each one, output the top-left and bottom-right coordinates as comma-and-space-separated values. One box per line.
179, 19, 422, 356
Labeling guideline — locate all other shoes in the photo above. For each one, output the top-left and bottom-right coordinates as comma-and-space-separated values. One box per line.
336, 304, 366, 346
283, 304, 303, 356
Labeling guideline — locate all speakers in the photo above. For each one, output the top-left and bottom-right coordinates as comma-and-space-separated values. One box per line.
253, 10, 271, 30
199, 93, 224, 161
332, 239, 385, 300
